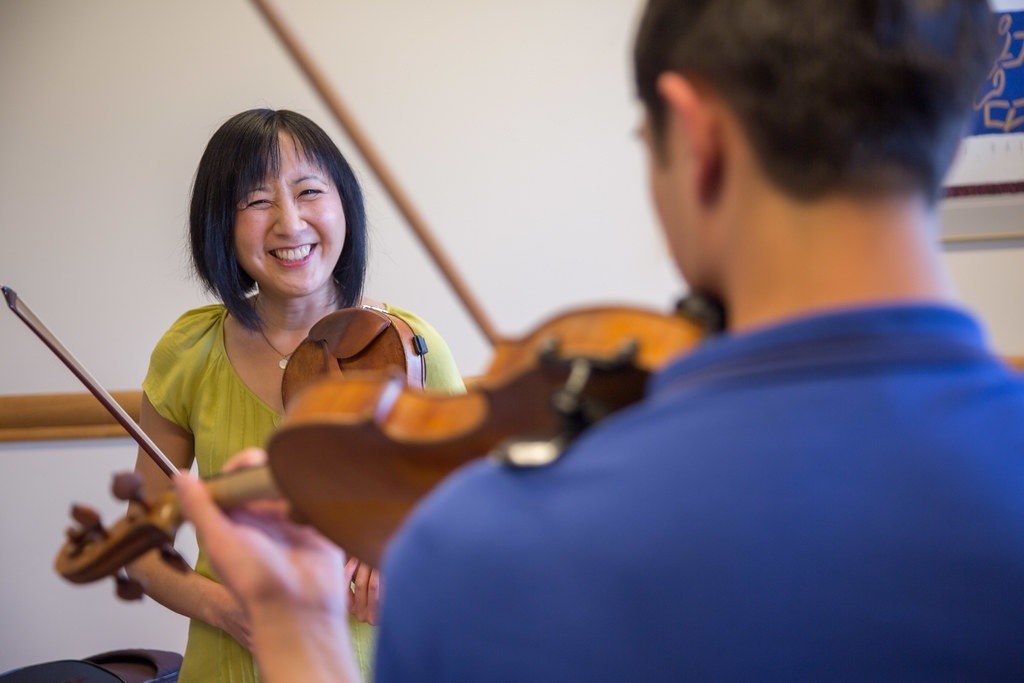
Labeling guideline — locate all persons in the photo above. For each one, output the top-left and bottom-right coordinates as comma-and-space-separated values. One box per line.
122, 109, 467, 683
168, 0, 1023, 683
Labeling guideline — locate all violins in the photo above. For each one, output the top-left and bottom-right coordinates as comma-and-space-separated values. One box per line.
54, 301, 725, 602
281, 304, 428, 416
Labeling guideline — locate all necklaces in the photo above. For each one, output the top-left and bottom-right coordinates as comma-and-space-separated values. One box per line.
255, 293, 292, 369
